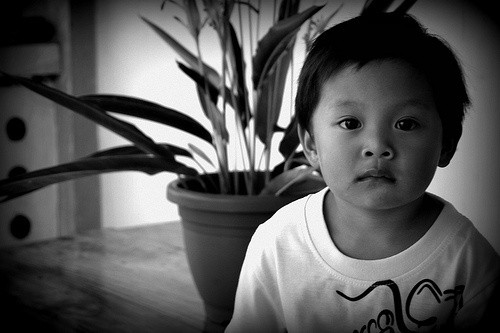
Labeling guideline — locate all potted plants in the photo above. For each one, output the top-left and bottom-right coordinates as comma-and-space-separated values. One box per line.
0, 0, 415, 306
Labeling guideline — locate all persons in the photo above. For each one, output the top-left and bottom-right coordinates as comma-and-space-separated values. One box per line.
223, 12, 500, 333
